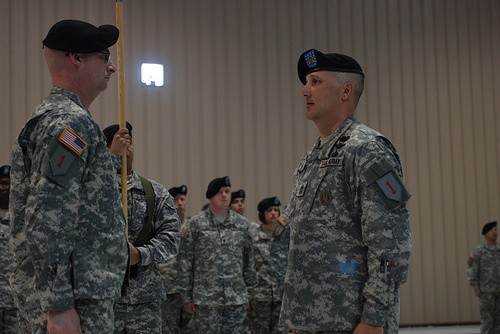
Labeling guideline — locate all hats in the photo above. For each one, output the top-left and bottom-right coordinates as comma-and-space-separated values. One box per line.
168, 185, 187, 198
205, 176, 231, 198
258, 196, 281, 211
231, 189, 245, 202
482, 221, 498, 235
42, 19, 119, 54
0, 165, 11, 178
102, 121, 133, 146
297, 49, 364, 86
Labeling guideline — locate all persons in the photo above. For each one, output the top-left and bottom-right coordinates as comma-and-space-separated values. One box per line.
230, 189, 246, 215
0, 166, 18, 334
162, 184, 188, 334
102, 121, 177, 334
277, 48, 413, 334
10, 19, 129, 334
247, 196, 290, 334
178, 176, 255, 334
200, 204, 212, 211
466, 220, 500, 334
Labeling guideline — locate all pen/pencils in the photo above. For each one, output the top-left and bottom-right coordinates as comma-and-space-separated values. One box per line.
380, 259, 397, 268
